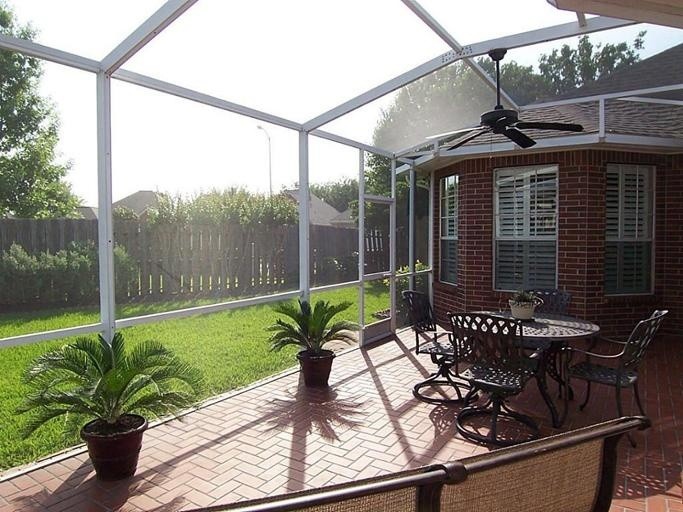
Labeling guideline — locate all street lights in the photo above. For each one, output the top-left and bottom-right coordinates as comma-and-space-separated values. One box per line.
256, 125, 274, 198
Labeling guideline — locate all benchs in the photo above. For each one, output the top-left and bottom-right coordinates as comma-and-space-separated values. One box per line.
133, 415, 653, 511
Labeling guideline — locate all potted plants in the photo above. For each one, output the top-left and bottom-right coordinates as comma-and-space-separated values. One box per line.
508, 290, 538, 319
11, 330, 206, 482
263, 296, 362, 387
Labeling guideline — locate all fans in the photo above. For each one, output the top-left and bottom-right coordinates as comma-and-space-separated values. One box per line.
442, 47, 585, 160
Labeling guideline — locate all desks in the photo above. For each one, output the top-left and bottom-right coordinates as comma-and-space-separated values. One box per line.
447, 312, 601, 426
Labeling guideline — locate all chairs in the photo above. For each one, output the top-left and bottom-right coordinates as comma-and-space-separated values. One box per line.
529, 291, 569, 354
402, 288, 479, 401
448, 310, 547, 445
560, 310, 668, 447
